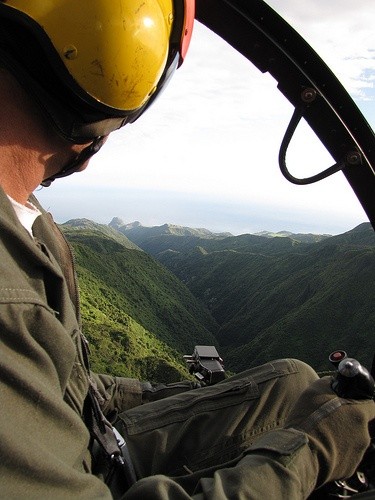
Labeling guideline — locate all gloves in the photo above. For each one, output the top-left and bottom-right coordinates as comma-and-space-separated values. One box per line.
284, 374, 375, 492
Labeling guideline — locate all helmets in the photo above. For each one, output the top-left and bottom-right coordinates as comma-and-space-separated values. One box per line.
4, 0, 199, 115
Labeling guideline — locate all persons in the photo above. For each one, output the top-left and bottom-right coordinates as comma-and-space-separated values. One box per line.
0, 0, 375, 500
